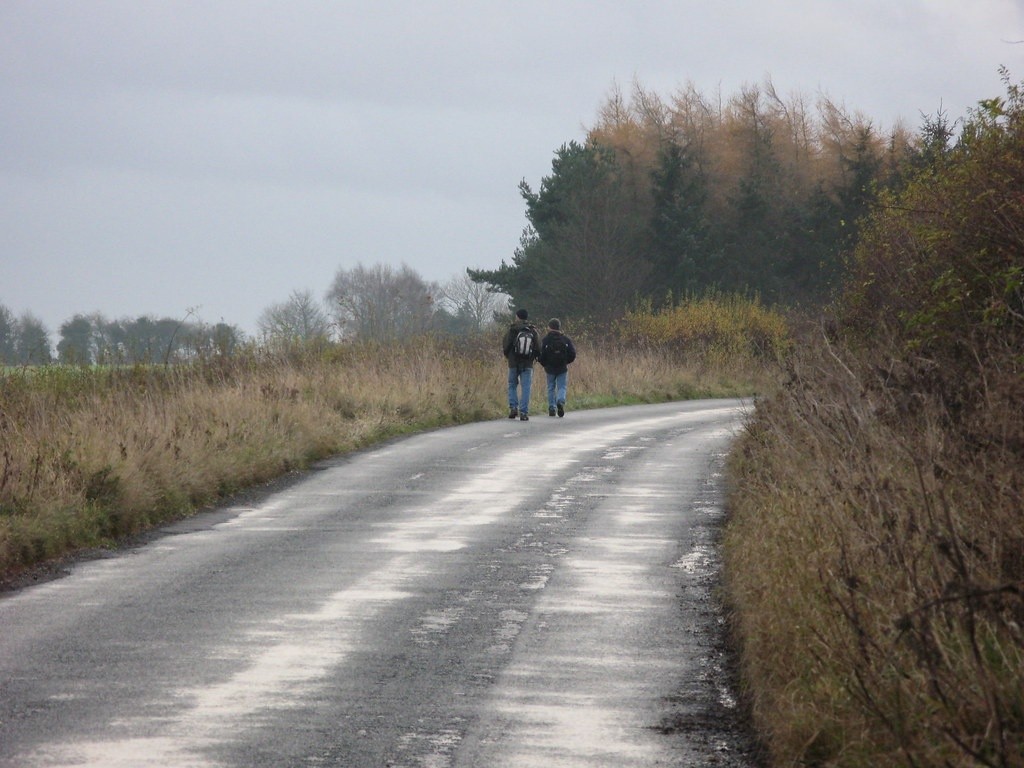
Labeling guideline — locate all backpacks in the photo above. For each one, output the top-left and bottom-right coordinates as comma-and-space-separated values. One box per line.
512, 324, 536, 359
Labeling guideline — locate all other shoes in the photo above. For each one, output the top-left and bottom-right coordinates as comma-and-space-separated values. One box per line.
509, 407, 518, 419
520, 412, 528, 420
549, 408, 556, 416
557, 402, 564, 417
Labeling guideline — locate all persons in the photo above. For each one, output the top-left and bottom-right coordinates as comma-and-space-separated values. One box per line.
502, 308, 542, 421
537, 317, 576, 417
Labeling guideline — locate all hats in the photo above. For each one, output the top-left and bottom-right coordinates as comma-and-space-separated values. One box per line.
548, 318, 561, 330
516, 309, 528, 319
